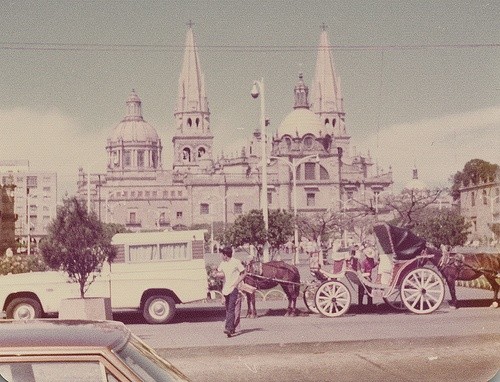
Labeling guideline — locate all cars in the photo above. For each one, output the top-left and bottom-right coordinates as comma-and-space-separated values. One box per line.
0, 319, 192, 382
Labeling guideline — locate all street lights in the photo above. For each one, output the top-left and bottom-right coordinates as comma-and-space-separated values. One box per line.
330, 196, 361, 249
144, 209, 170, 232
479, 194, 500, 223
361, 190, 393, 261
106, 189, 124, 223
267, 154, 320, 266
209, 192, 239, 254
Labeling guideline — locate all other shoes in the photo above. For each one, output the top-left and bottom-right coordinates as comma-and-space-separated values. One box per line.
224, 330, 232, 337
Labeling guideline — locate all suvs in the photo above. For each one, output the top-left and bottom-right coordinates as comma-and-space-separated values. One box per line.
0, 229, 209, 325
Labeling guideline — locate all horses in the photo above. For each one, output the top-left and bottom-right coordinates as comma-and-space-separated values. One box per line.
421, 247, 500, 309
240, 261, 300, 318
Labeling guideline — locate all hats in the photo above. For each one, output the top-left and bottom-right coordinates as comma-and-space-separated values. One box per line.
363, 248, 375, 258
440, 243, 456, 254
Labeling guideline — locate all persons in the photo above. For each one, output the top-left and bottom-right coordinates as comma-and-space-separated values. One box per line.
218, 247, 247, 337
334, 241, 360, 274
358, 248, 378, 305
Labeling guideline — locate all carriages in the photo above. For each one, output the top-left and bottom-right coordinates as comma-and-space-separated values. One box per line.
240, 223, 445, 319
